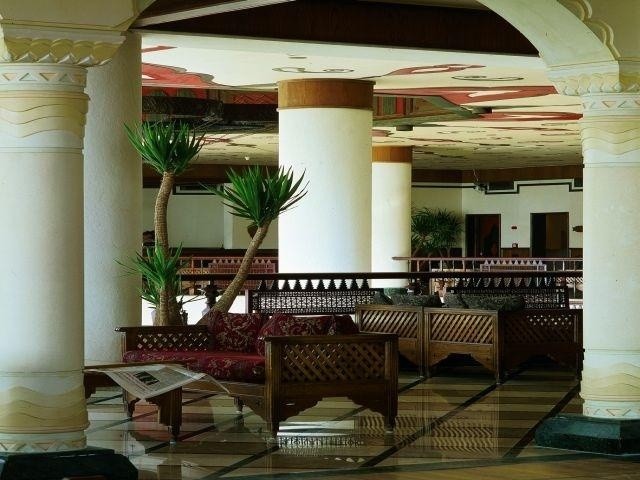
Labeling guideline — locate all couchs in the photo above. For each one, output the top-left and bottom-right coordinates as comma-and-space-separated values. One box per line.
114, 306, 403, 440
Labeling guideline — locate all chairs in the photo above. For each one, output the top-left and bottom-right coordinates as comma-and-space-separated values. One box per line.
355, 302, 583, 385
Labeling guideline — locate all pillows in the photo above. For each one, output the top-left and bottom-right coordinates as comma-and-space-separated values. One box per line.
373, 289, 527, 310
205, 309, 356, 358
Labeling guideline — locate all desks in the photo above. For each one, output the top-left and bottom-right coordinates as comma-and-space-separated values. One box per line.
83, 358, 198, 441
